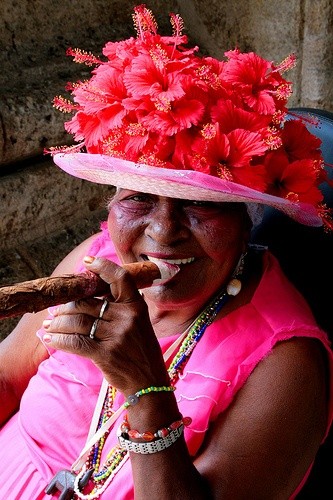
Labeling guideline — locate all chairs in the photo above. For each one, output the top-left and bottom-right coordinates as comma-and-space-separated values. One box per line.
245, 107, 333, 500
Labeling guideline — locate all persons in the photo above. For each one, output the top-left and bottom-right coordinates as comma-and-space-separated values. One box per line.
0, 3, 333, 500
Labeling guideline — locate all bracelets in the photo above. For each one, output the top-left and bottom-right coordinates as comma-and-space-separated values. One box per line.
123, 384, 176, 409
116, 414, 192, 455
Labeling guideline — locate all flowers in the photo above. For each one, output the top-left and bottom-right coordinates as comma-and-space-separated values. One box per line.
43, 5, 333, 230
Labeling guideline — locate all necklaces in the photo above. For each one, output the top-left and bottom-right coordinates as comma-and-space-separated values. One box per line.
44, 245, 252, 500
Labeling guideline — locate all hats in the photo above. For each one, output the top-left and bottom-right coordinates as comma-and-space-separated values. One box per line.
43, 3, 331, 231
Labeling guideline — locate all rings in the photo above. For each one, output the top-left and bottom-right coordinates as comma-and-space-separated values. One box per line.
90, 318, 99, 339
99, 300, 107, 318
